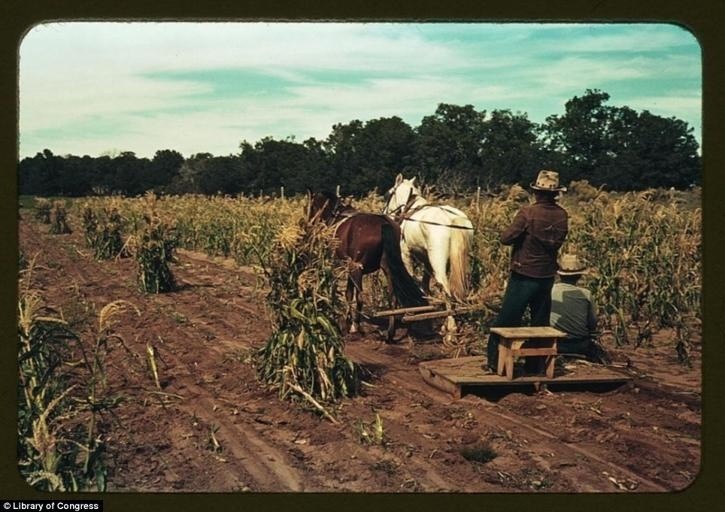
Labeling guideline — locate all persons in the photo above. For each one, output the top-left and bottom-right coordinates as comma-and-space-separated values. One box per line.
481, 169, 569, 373
548, 254, 603, 361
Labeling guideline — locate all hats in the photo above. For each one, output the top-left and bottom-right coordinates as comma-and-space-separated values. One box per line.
530, 170, 568, 193
556, 254, 590, 276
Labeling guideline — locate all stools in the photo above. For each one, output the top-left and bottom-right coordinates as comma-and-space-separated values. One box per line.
489, 326, 568, 381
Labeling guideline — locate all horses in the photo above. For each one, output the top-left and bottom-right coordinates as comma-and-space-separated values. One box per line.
303, 185, 433, 344
383, 171, 476, 344
